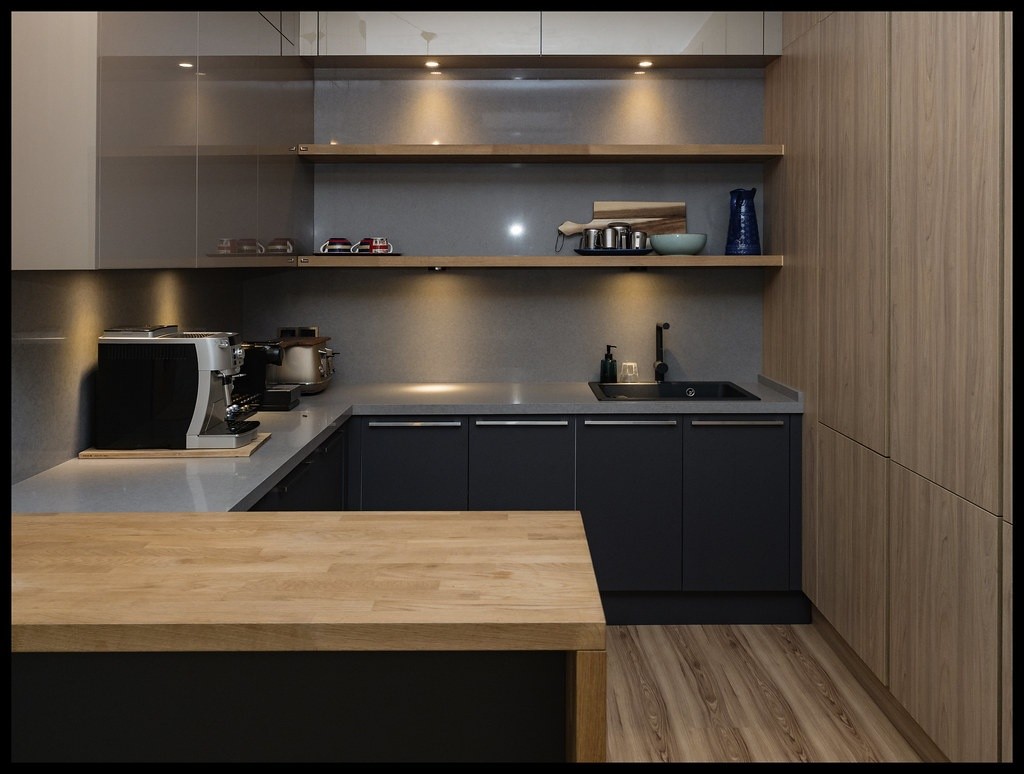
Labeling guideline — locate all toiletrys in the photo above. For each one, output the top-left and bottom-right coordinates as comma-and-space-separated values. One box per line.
599, 343, 618, 383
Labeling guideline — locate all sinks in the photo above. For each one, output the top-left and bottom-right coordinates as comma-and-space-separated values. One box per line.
588, 378, 760, 403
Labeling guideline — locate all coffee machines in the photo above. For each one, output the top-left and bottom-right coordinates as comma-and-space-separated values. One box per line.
96, 324, 261, 447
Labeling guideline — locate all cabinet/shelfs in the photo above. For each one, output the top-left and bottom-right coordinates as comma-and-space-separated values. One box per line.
11, 510, 607, 764
351, 413, 804, 593
254, 420, 352, 512
298, 144, 784, 267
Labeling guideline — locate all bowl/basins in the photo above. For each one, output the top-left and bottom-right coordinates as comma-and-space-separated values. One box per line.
650, 233, 707, 256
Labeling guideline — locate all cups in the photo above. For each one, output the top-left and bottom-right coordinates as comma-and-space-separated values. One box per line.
620, 362, 638, 383
217, 238, 237, 253
372, 238, 393, 253
579, 228, 602, 250
351, 238, 374, 253
631, 232, 647, 250
266, 238, 295, 253
607, 222, 632, 249
237, 238, 264, 254
594, 228, 618, 248
320, 237, 353, 252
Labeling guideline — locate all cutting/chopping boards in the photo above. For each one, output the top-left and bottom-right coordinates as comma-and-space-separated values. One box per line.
78, 431, 272, 459
558, 200, 687, 255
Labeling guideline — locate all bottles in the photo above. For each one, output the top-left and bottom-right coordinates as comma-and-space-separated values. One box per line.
726, 188, 761, 256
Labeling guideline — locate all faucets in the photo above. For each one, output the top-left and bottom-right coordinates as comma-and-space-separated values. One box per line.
653, 321, 670, 383
